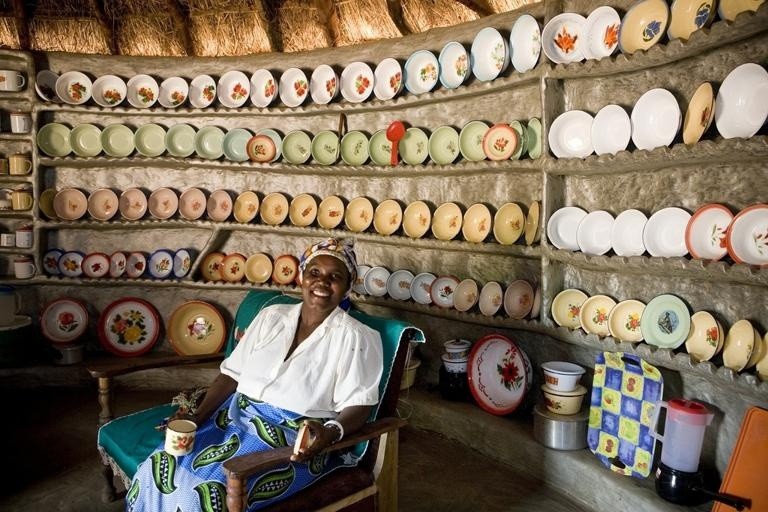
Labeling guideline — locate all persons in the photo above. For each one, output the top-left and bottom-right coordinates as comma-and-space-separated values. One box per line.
124, 237, 386, 510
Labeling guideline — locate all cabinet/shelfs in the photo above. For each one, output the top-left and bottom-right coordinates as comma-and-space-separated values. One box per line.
1, 47, 39, 285
27, 0, 767, 412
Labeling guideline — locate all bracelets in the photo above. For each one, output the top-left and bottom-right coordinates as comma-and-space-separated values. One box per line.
324, 419, 344, 445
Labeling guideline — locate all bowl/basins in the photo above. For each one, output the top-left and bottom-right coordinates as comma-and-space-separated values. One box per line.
541, 360, 584, 391
98, 296, 161, 359
400, 358, 421, 390
465, 332, 535, 418
439, 353, 471, 374
542, 386, 589, 415
40, 298, 87, 346
444, 336, 472, 361
166, 299, 228, 358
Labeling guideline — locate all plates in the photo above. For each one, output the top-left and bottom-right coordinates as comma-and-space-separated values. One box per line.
42, 249, 547, 320
40, 188, 541, 247
547, 61, 768, 161
541, 0, 765, 71
544, 201, 768, 265
35, 116, 544, 166
34, 12, 541, 106
549, 286, 768, 380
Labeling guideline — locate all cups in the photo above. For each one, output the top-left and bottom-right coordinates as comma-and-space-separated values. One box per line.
0, 286, 18, 325
642, 394, 718, 475
0, 69, 36, 281
161, 417, 198, 457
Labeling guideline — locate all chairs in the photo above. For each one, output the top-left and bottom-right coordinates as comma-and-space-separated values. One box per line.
87, 287, 423, 511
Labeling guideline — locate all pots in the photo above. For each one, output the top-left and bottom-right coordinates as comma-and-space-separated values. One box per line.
526, 400, 594, 450
652, 462, 746, 512
